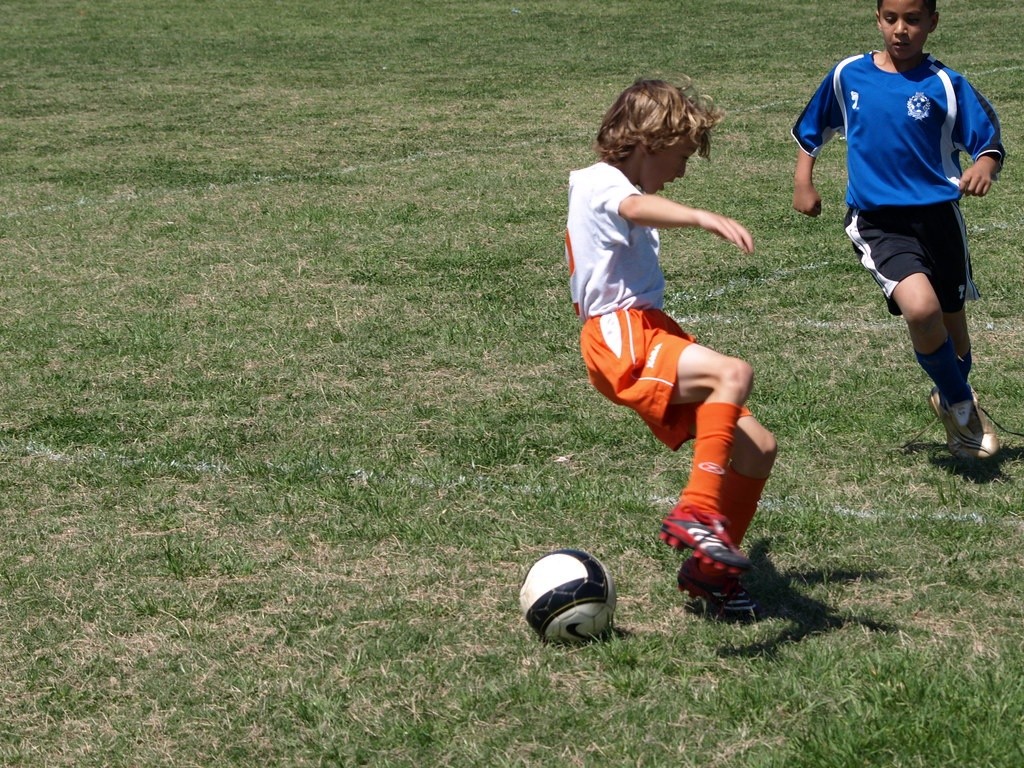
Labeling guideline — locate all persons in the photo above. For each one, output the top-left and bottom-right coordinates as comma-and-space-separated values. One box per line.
791, 0, 1005, 462
565, 73, 777, 616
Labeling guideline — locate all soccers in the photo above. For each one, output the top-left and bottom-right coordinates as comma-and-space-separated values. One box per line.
517, 549, 619, 647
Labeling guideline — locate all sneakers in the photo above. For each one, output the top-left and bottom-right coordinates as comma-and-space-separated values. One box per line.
677, 557, 763, 621
659, 503, 752, 576
897, 384, 1024, 460
